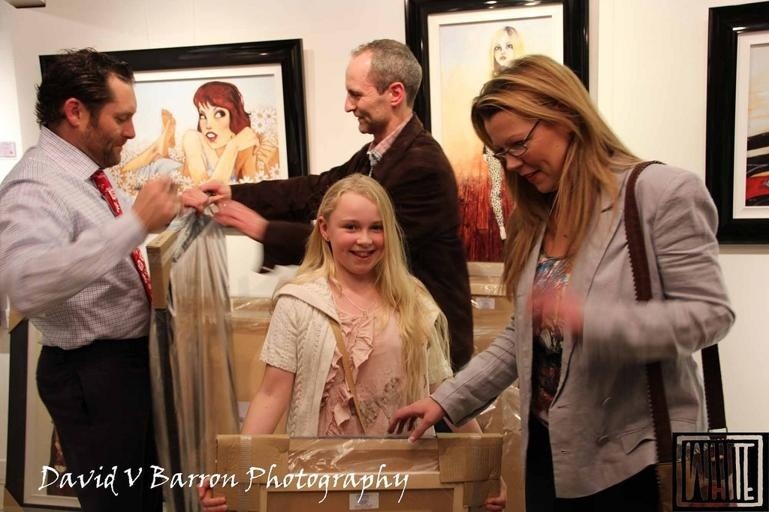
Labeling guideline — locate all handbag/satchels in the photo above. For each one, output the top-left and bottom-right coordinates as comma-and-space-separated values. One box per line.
657, 439, 738, 511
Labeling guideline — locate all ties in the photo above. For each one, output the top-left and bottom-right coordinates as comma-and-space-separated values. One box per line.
90, 168, 152, 307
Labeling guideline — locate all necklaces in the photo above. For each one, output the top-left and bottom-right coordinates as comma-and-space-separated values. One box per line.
341, 292, 369, 315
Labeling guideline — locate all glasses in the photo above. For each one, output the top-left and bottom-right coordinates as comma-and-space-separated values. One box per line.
492, 117, 543, 163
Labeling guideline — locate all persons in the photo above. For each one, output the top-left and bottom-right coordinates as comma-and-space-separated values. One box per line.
193, 169, 510, 511
386, 51, 736, 511
2, 44, 216, 511
192, 38, 478, 368
119, 80, 280, 189
479, 24, 530, 247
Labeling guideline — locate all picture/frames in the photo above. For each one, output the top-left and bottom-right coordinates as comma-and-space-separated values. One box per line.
404, 1, 589, 278
705, 1, 768, 245
146, 190, 241, 512
3, 300, 83, 512
214, 433, 503, 512
39, 39, 307, 284
